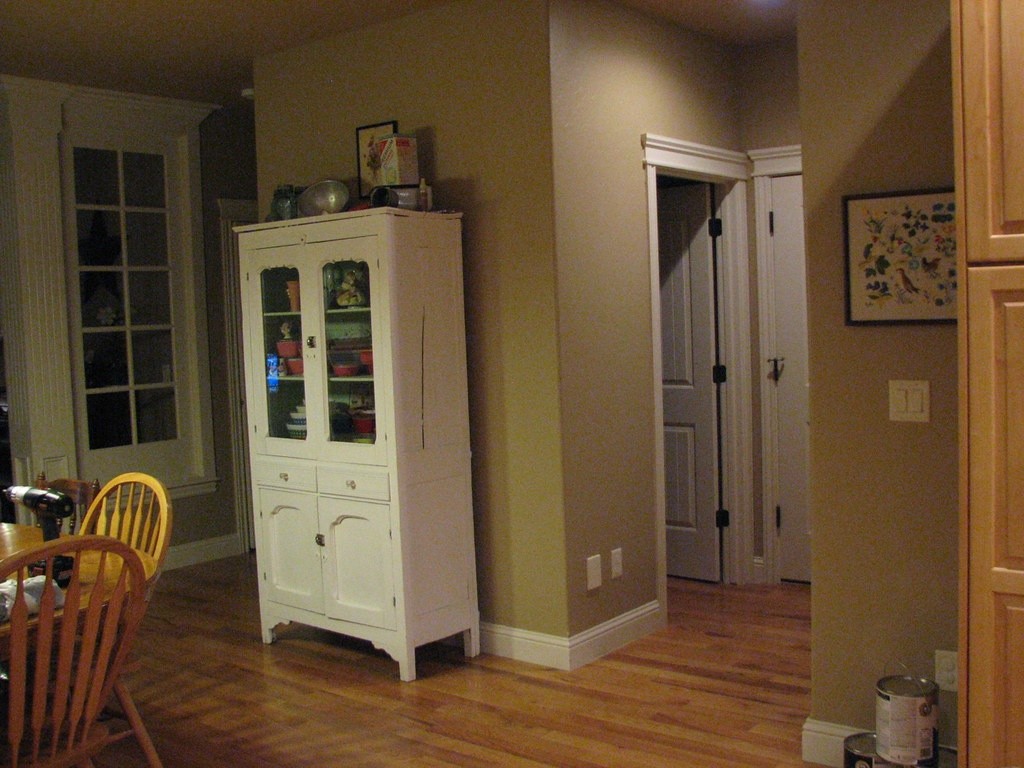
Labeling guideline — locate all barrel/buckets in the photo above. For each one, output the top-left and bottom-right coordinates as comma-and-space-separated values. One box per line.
876, 660, 939, 765
844, 732, 889, 768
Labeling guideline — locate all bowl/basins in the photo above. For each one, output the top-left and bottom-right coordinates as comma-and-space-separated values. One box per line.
298, 181, 350, 215
352, 418, 375, 433
287, 358, 304, 376
333, 364, 359, 376
286, 406, 307, 439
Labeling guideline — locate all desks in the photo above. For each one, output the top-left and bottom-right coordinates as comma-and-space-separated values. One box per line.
0, 522, 162, 721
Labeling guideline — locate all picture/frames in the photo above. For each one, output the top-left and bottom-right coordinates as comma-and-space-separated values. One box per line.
841, 186, 959, 326
355, 121, 398, 199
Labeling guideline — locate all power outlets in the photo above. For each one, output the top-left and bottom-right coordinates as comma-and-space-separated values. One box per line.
586, 554, 602, 591
611, 548, 623, 580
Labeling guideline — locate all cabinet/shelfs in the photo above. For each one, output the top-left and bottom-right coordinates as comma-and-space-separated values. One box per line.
1, 73, 222, 507
232, 206, 478, 682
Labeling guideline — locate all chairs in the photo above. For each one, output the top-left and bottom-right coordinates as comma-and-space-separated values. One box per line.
0, 474, 171, 768
